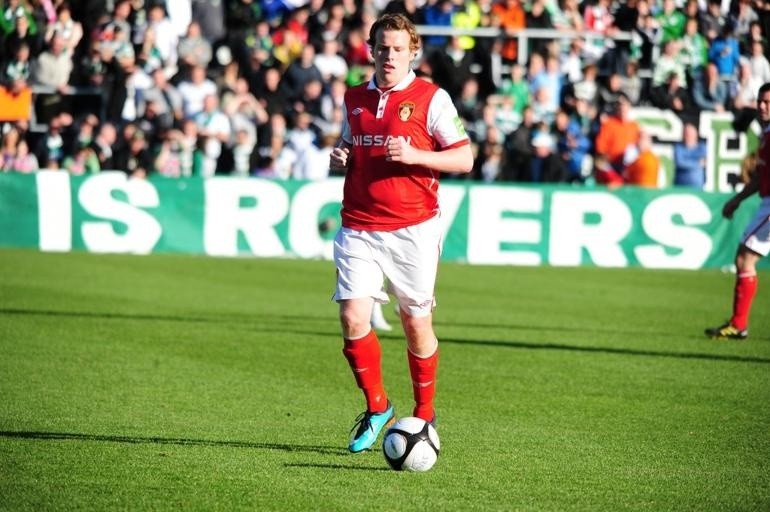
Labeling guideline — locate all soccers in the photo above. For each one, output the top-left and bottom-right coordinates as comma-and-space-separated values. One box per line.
383, 417, 440, 472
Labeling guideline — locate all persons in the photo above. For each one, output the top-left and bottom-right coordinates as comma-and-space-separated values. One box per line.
322, 7, 472, 453
702, 83, 770, 340
0, 1, 769, 193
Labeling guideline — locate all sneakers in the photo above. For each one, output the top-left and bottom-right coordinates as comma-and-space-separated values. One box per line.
347, 396, 396, 453
704, 322, 749, 340
